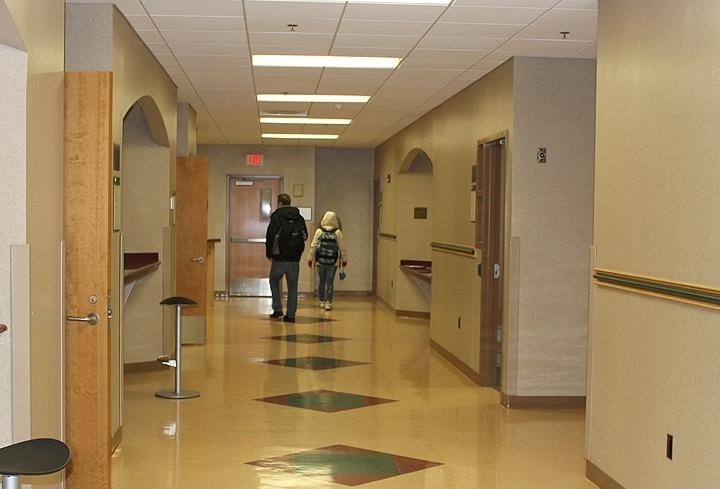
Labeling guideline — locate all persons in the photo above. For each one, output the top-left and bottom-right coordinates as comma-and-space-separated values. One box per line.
308, 210, 347, 309
266, 194, 308, 322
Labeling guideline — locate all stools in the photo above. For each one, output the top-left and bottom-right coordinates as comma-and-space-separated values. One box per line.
154, 296, 201, 401
0, 438, 72, 488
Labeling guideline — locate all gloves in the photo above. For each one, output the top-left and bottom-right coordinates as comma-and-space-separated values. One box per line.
342, 261, 347, 267
307, 261, 313, 268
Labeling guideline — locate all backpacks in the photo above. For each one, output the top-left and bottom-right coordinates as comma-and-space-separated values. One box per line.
315, 224, 339, 266
274, 211, 304, 257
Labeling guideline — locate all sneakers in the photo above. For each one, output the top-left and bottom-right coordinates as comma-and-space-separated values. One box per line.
283, 315, 295, 323
324, 302, 333, 311
269, 312, 283, 318
319, 300, 325, 308
